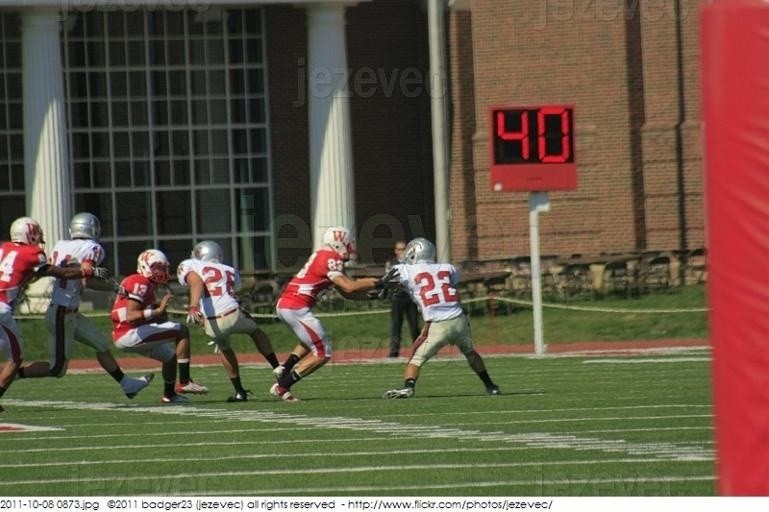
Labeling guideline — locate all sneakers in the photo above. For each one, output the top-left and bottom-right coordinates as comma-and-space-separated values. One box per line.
270, 365, 300, 401
382, 387, 414, 399
226, 390, 247, 402
176, 380, 208, 394
487, 384, 499, 395
126, 373, 154, 399
162, 393, 188, 403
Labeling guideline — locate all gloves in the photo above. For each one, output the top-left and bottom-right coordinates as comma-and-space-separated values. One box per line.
368, 267, 401, 300
93, 266, 112, 280
184, 304, 203, 324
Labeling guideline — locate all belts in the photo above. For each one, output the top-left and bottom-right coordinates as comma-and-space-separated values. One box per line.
66, 307, 78, 313
207, 308, 236, 320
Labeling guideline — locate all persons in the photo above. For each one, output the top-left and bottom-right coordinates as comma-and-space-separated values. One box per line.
268, 226, 400, 403
381, 238, 499, 398
110, 249, 209, 403
377, 242, 420, 357
0, 213, 155, 399
0, 216, 110, 412
177, 240, 278, 403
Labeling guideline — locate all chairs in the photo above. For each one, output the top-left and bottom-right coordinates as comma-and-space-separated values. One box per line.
160, 244, 708, 320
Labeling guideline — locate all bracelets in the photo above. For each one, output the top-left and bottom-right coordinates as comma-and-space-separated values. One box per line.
143, 309, 153, 322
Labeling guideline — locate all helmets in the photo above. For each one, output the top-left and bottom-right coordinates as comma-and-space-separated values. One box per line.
136, 249, 170, 283
10, 217, 46, 245
403, 237, 436, 265
68, 212, 103, 239
323, 227, 355, 261
192, 240, 225, 263
